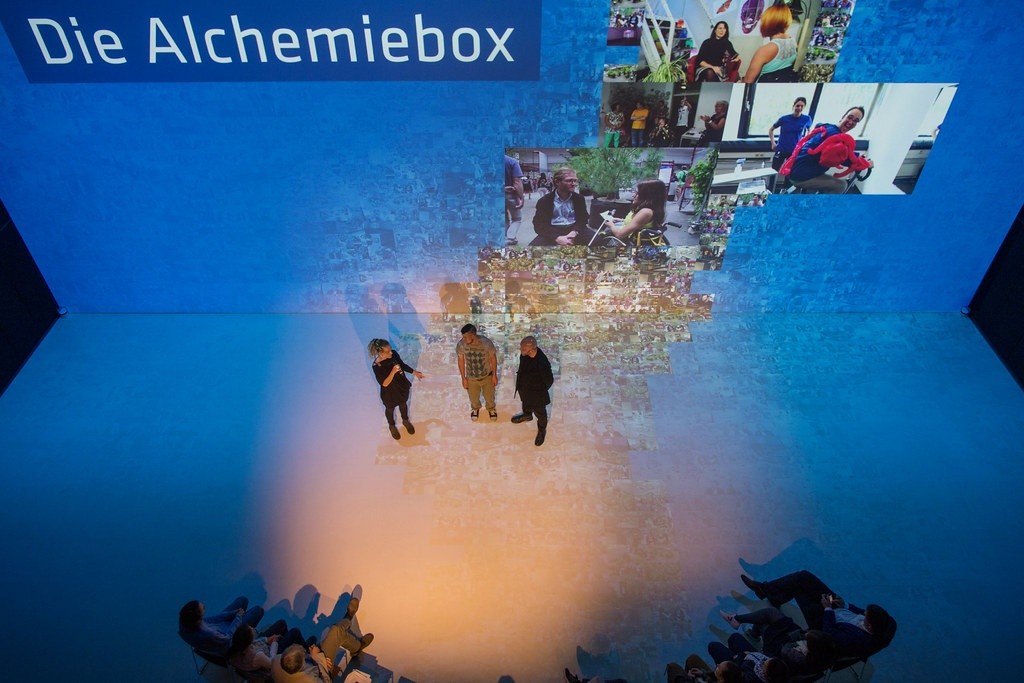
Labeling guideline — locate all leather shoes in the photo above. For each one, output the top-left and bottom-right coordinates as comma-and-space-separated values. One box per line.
741, 574, 767, 600
511, 414, 533, 423
535, 430, 546, 446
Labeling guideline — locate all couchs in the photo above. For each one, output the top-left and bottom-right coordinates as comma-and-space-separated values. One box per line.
687, 55, 742, 82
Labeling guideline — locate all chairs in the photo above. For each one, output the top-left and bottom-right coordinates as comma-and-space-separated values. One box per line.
177, 632, 235, 683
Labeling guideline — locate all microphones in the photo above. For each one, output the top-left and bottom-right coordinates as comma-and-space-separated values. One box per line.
392, 359, 404, 376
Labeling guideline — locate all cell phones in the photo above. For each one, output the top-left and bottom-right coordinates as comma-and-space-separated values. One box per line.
827, 593, 832, 604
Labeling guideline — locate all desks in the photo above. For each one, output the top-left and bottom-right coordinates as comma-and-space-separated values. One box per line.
679, 127, 703, 147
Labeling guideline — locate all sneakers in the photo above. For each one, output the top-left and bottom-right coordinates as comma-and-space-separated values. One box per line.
743, 625, 761, 642
489, 409, 497, 421
564, 667, 578, 683
719, 609, 740, 631
471, 409, 479, 421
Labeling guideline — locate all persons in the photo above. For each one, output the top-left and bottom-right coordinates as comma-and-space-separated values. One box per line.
815, 31, 824, 47
702, 222, 727, 234
178, 597, 374, 683
604, 179, 667, 247
628, 12, 638, 28
456, 324, 498, 421
694, 21, 740, 82
665, 570, 898, 683
549, 177, 553, 192
826, 34, 837, 46
823, 0, 852, 9
786, 106, 874, 194
696, 101, 728, 147
603, 102, 625, 148
747, 194, 767, 207
504, 147, 524, 245
368, 339, 425, 440
537, 172, 548, 198
706, 208, 732, 220
677, 166, 688, 200
528, 168, 598, 245
512, 336, 554, 446
564, 667, 628, 683
534, 261, 549, 270
616, 14, 627, 28
768, 98, 812, 192
742, 4, 797, 84
675, 96, 692, 147
630, 101, 649, 147
648, 100, 676, 148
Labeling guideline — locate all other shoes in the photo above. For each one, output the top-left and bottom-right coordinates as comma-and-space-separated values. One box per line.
344, 598, 359, 620
389, 425, 400, 440
402, 420, 414, 435
506, 237, 518, 245
350, 633, 374, 657
